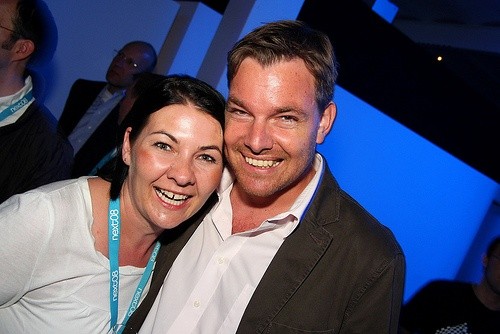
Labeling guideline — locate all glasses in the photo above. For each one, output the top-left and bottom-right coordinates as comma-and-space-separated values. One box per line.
114, 50, 145, 73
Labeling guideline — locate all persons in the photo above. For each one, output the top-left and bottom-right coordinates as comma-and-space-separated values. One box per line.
0, 0, 75, 207
123, 19, 408, 334
58, 41, 158, 176
398, 233, 500, 333
0, 73, 226, 334
